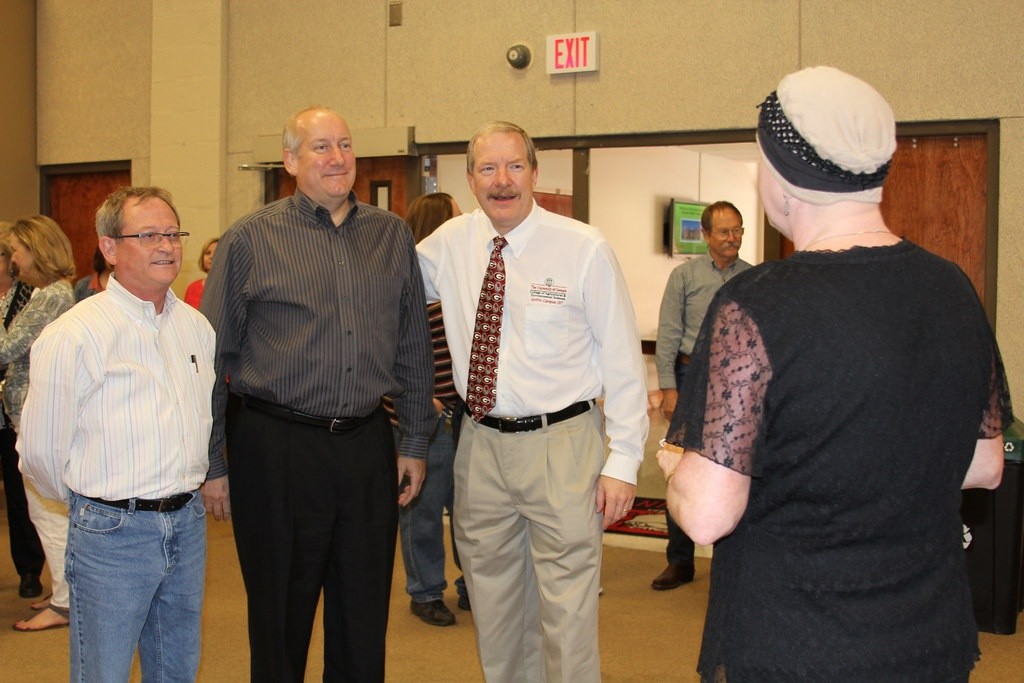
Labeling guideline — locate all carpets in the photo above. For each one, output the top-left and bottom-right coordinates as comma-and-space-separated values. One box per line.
603, 495, 674, 538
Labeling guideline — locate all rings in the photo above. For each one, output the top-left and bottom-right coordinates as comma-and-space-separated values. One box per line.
623, 509, 628, 512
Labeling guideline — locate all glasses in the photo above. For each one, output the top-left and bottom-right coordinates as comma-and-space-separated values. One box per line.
110, 231, 190, 246
708, 228, 744, 240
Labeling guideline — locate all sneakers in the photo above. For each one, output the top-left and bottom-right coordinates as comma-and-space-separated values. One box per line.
458, 596, 471, 610
410, 599, 455, 626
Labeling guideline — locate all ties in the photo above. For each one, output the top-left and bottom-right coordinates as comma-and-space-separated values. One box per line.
466, 236, 508, 428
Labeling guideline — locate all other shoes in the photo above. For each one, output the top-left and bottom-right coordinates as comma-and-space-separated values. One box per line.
20, 573, 42, 597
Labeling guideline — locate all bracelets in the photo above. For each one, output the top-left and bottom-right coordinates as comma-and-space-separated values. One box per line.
665, 470, 674, 488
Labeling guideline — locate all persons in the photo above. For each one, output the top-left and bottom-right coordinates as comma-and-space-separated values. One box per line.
414, 121, 651, 683
655, 64, 1016, 683
0, 214, 76, 631
16, 185, 216, 683
184, 237, 220, 310
73, 246, 115, 303
651, 202, 755, 589
197, 107, 438, 683
383, 193, 471, 625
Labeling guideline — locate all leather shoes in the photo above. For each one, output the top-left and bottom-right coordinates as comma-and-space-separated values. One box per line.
651, 562, 694, 590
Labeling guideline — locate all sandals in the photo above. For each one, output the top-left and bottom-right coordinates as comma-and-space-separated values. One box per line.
31, 592, 53, 610
13, 603, 70, 632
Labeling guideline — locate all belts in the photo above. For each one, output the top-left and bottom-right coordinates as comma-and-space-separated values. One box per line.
227, 392, 384, 434
69, 487, 201, 513
679, 353, 690, 365
463, 397, 596, 432
